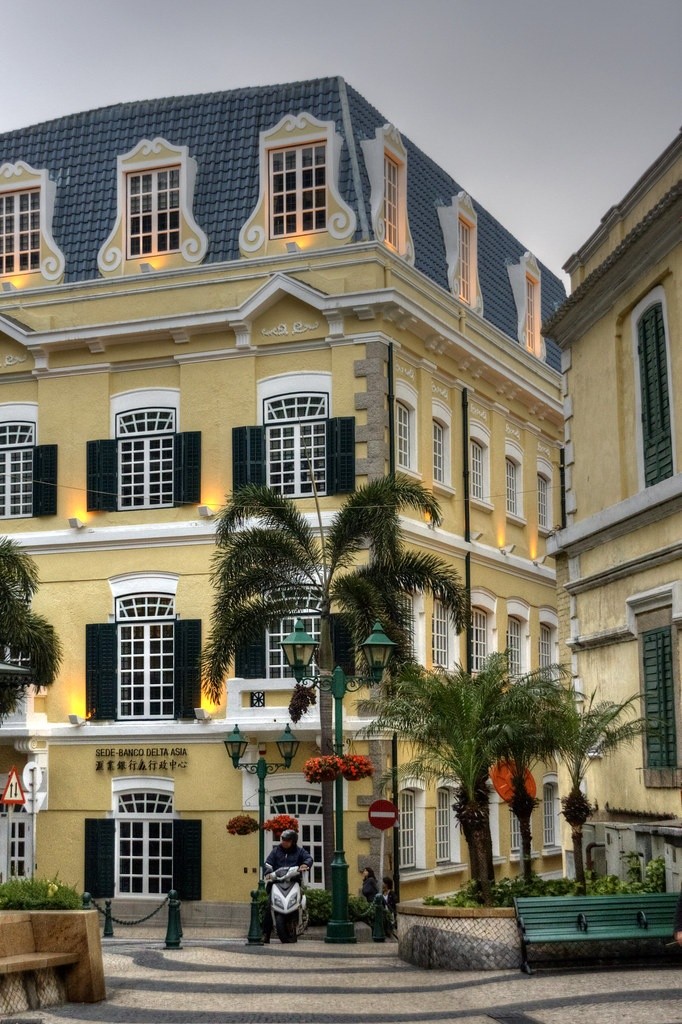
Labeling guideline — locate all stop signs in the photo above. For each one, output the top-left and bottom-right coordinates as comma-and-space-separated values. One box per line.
368, 799, 398, 831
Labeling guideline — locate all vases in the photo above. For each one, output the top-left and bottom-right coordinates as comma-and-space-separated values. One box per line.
237, 830, 251, 835
273, 828, 285, 835
319, 774, 338, 781
344, 774, 362, 781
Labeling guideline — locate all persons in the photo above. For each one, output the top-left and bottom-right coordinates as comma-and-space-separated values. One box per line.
379, 876, 399, 918
261, 828, 314, 933
360, 866, 379, 911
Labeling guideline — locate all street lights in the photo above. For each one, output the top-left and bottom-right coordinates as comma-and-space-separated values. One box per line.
223, 722, 302, 947
277, 615, 399, 943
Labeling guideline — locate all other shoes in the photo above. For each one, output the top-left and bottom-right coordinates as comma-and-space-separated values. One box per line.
261, 934, 270, 943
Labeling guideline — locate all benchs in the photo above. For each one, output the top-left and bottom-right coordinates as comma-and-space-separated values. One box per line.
0, 911, 106, 1016
510, 892, 682, 974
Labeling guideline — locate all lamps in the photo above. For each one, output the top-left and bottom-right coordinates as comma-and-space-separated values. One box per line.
194, 708, 212, 721
69, 517, 85, 528
499, 542, 516, 556
470, 531, 483, 541
198, 506, 217, 517
2, 281, 18, 291
426, 516, 444, 529
68, 714, 87, 727
140, 263, 157, 274
533, 555, 547, 567
285, 241, 303, 253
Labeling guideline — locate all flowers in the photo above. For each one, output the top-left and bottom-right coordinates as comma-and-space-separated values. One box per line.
341, 754, 374, 778
226, 814, 259, 834
262, 815, 299, 833
303, 755, 340, 783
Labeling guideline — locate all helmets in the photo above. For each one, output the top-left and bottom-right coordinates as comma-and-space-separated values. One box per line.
280, 829, 299, 843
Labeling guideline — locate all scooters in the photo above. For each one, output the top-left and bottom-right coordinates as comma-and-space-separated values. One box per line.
263, 862, 310, 943
